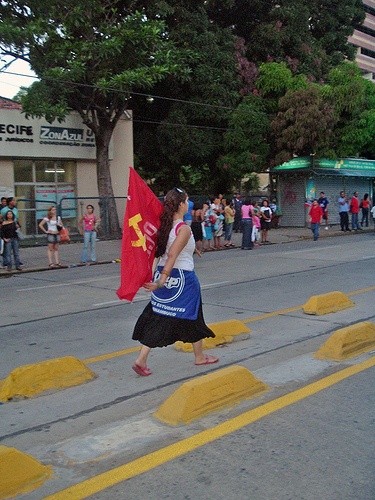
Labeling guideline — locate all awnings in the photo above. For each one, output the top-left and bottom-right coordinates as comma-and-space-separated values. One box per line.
312, 169, 375, 176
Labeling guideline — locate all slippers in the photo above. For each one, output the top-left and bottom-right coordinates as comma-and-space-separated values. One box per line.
131, 366, 152, 376
192, 354, 219, 365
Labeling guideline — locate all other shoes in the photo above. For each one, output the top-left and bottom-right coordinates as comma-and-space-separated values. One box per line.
2, 262, 23, 267
49, 263, 63, 268
202, 243, 235, 252
7, 266, 22, 271
260, 240, 270, 243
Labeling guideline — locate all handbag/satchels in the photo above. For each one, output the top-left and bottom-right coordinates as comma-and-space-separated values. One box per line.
59, 229, 70, 242
57, 226, 62, 231
274, 207, 283, 217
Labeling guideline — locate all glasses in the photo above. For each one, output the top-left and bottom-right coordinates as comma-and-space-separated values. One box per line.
173, 186, 186, 194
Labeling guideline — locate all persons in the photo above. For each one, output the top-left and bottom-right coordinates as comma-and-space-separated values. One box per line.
251, 201, 262, 243
0, 197, 24, 270
361, 193, 371, 226
319, 192, 330, 230
81, 205, 98, 262
131, 188, 219, 375
240, 198, 254, 249
351, 192, 362, 230
371, 206, 375, 224
309, 199, 322, 241
339, 192, 351, 231
183, 193, 236, 253
40, 206, 63, 266
260, 200, 272, 242
232, 191, 244, 233
270, 198, 280, 229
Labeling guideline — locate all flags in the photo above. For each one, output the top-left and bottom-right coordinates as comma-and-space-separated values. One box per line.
116, 167, 165, 301
